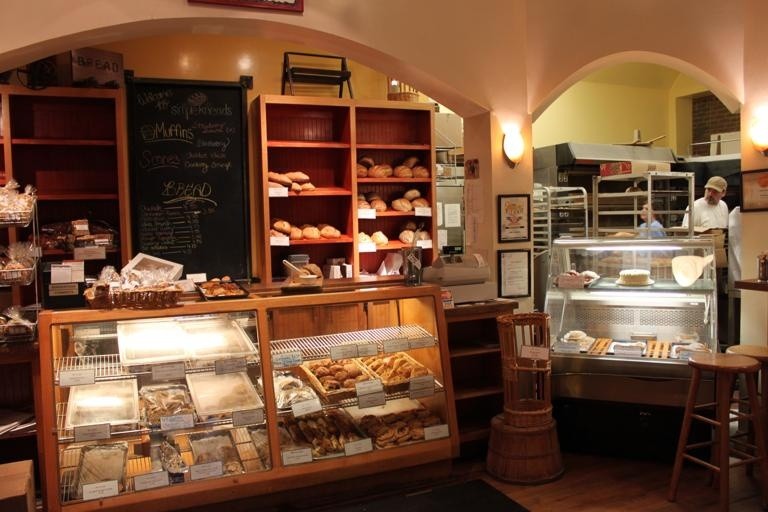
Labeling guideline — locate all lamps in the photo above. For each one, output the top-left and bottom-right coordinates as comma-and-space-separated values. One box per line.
748, 104, 767, 157
502, 123, 528, 168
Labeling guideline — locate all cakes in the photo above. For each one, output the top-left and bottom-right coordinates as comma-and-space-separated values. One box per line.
620, 269, 650, 285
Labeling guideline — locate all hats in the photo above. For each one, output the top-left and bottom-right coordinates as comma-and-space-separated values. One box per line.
704, 175, 727, 193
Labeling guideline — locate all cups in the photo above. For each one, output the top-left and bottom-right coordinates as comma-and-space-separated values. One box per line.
403, 246, 423, 285
759, 259, 768, 282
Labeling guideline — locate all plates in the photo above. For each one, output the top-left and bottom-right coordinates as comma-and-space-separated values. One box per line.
607, 341, 647, 355
669, 343, 711, 359
563, 330, 586, 340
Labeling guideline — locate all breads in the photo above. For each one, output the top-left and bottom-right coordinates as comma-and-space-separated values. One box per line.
267, 168, 315, 192
560, 329, 595, 352
83, 267, 174, 298
358, 156, 428, 178
0, 244, 36, 285
271, 217, 341, 240
357, 222, 433, 247
0, 318, 32, 342
358, 190, 429, 212
556, 269, 598, 285
278, 352, 441, 458
0, 183, 33, 224
203, 275, 244, 297
145, 392, 196, 425
293, 263, 323, 282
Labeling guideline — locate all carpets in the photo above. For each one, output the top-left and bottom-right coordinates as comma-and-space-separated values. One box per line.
357, 476, 528, 512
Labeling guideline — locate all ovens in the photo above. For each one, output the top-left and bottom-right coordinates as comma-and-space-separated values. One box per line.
533, 143, 676, 257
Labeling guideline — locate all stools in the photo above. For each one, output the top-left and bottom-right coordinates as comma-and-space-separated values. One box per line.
718, 343, 767, 477
666, 352, 767, 511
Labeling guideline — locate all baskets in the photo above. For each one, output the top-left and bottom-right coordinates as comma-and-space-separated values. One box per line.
82, 283, 183, 310
298, 357, 379, 403
356, 351, 436, 394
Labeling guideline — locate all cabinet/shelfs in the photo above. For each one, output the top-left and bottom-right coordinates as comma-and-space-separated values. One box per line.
0, 82, 131, 306
251, 89, 354, 288
351, 98, 438, 284
592, 170, 696, 236
39, 290, 452, 512
542, 239, 715, 407
441, 300, 519, 475
0, 189, 41, 348
532, 185, 588, 247
0, 344, 48, 512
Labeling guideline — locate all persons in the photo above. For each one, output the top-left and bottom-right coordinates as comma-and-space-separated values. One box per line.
637, 203, 667, 237
681, 175, 728, 273
726, 203, 740, 299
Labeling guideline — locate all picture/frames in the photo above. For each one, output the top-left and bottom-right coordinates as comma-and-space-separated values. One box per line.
496, 248, 532, 299
738, 166, 768, 214
496, 192, 532, 244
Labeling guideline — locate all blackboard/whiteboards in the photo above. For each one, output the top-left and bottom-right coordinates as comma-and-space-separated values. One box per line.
123, 68, 252, 285
